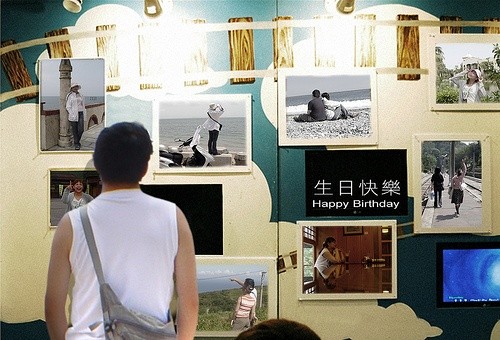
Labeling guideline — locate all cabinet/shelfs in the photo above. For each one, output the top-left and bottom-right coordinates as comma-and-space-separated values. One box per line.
377, 225, 392, 294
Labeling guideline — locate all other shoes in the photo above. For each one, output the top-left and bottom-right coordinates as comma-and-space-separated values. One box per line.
75, 143, 81, 150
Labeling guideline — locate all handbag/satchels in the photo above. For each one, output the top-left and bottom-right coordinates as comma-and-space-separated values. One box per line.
99, 283, 177, 340
219, 124, 222, 130
430, 190, 433, 199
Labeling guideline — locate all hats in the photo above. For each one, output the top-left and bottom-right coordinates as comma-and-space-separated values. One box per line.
471, 69, 481, 78
208, 103, 217, 110
71, 81, 81, 89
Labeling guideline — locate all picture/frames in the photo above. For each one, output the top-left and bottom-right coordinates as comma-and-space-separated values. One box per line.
428, 33, 500, 111
342, 225, 364, 236
152, 93, 252, 174
296, 219, 398, 301
412, 133, 491, 234
278, 68, 379, 146
173, 256, 277, 337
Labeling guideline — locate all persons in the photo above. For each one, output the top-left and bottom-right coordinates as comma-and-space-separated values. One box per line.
451, 157, 466, 217
314, 237, 339, 267
316, 265, 340, 290
321, 93, 359, 120
229, 278, 257, 331
203, 103, 224, 155
450, 69, 486, 103
430, 167, 444, 208
45, 122, 199, 340
61, 179, 94, 213
65, 82, 86, 150
293, 90, 326, 122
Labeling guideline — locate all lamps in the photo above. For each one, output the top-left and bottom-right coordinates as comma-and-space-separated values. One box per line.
143, 0, 163, 19
335, 0, 356, 15
62, 0, 83, 14
